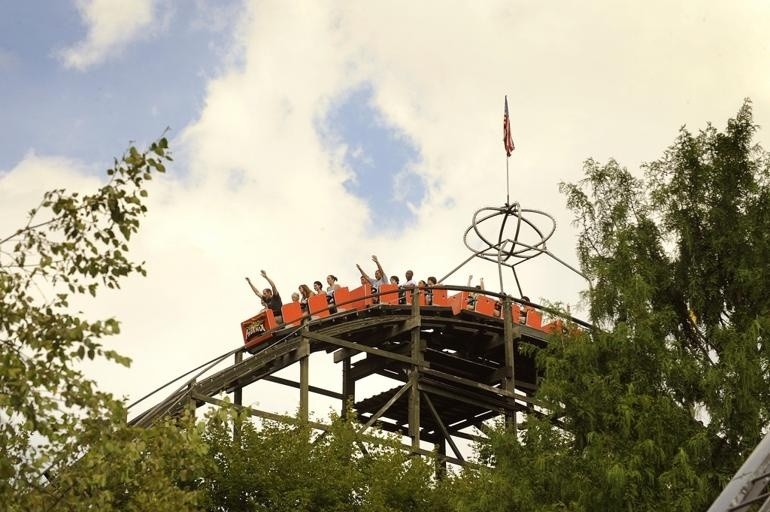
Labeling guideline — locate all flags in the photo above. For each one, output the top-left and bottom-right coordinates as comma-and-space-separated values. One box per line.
502, 95, 515, 159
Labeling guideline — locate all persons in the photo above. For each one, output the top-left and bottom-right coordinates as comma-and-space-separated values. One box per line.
314, 281, 328, 296
467, 275, 486, 310
424, 276, 443, 297
494, 291, 508, 317
245, 269, 283, 325
398, 270, 416, 296
417, 280, 427, 293
326, 275, 341, 292
355, 255, 389, 302
298, 284, 316, 305
360, 275, 369, 285
520, 296, 536, 324
390, 276, 399, 285
260, 298, 268, 314
291, 292, 309, 314
